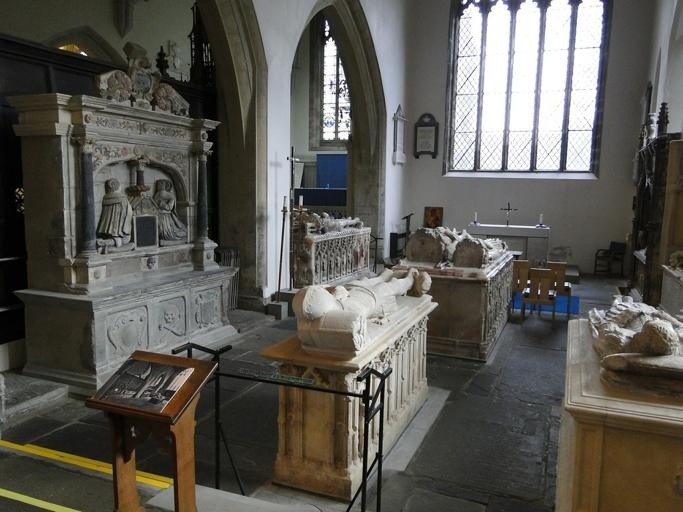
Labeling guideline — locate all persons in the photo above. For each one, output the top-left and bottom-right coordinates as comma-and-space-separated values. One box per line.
589, 292, 683, 361
290, 264, 421, 323
426, 209, 439, 227
96, 178, 133, 248
151, 178, 187, 241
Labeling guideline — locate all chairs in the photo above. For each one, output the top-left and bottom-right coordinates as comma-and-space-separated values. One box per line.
512, 259, 571, 319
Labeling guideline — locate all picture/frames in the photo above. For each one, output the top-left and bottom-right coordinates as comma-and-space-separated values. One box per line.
414, 113, 439, 159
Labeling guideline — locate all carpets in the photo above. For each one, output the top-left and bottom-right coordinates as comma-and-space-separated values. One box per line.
509, 293, 578, 313
